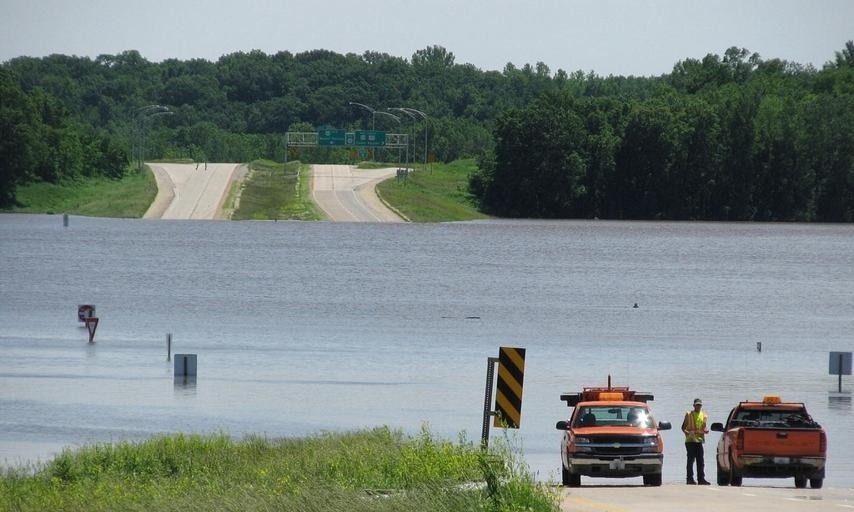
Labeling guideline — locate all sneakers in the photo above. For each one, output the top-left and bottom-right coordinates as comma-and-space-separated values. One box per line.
687, 479, 711, 485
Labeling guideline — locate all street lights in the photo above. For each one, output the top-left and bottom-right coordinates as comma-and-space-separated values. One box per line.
349, 102, 427, 172
132, 104, 174, 172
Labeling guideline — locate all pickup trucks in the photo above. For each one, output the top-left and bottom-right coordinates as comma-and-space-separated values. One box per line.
556, 374, 672, 487
710, 393, 828, 489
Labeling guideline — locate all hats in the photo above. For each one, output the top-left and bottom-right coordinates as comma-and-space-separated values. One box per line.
694, 398, 703, 406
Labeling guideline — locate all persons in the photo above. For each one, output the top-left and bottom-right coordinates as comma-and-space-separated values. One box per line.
680, 397, 711, 485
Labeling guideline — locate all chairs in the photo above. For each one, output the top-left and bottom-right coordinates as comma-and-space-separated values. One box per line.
583, 413, 594, 425
627, 409, 641, 424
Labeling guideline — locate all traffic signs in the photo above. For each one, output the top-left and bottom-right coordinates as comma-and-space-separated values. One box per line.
288, 129, 408, 148
